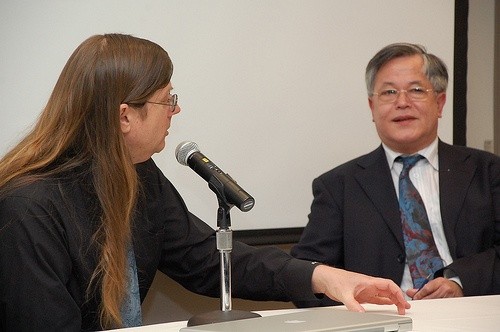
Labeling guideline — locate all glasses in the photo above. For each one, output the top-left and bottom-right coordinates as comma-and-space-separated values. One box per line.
144, 93, 178, 112
370, 85, 439, 104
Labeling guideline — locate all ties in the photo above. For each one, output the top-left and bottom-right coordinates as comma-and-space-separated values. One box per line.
394, 154, 444, 288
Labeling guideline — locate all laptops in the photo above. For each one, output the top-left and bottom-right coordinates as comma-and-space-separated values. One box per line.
178, 307, 413, 332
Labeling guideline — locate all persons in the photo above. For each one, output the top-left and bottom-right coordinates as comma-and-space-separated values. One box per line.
0, 33, 410, 332
289, 42, 499, 308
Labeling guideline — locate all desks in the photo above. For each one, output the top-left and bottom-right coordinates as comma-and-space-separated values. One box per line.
97, 295, 500, 332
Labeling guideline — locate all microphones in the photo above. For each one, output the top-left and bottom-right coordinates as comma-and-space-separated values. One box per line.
175, 140, 255, 213
